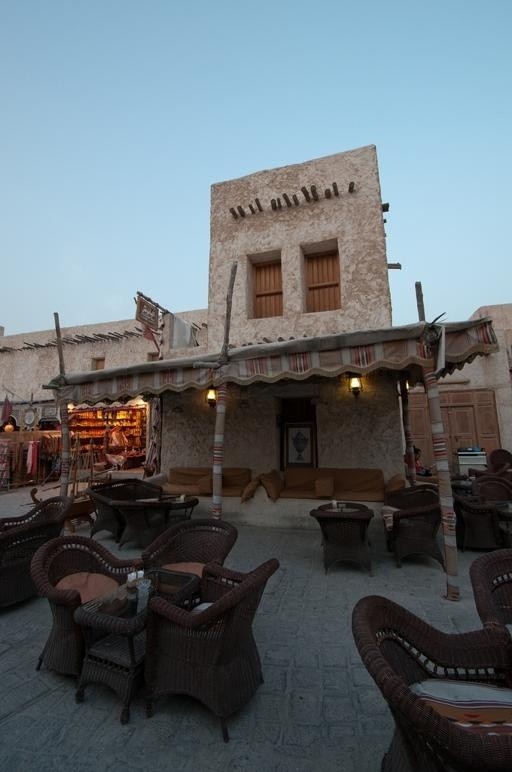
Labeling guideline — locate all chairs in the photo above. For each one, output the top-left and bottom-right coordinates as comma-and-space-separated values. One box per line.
310, 448, 512, 577
470, 548, 512, 634
352, 595, 512, 772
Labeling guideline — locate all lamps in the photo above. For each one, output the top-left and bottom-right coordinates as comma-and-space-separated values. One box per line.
205, 386, 217, 408
347, 373, 362, 398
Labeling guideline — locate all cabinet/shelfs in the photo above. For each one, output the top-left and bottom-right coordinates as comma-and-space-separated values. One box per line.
71, 411, 143, 456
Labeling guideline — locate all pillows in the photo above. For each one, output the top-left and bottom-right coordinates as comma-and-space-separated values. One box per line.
384, 475, 406, 494
262, 470, 284, 502
241, 473, 265, 503
197, 473, 213, 494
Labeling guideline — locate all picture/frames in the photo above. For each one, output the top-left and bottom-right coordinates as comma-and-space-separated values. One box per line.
283, 422, 316, 469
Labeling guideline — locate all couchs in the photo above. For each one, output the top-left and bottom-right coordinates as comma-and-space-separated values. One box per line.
278, 465, 385, 501
161, 466, 252, 497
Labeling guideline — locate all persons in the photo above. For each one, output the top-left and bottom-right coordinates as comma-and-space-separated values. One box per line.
414, 448, 432, 476
112, 426, 128, 446
